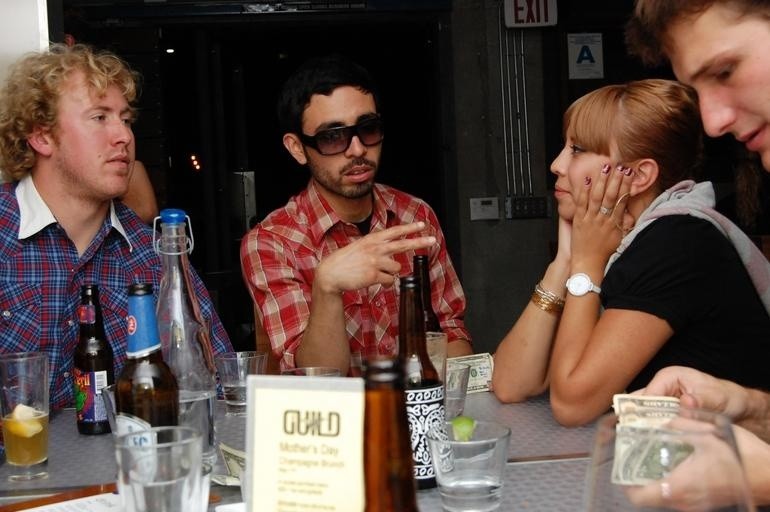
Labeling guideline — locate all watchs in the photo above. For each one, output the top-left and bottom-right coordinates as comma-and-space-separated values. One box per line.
566, 272, 601, 296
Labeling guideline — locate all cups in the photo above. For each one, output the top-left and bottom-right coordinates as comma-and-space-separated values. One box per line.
423, 416, 512, 512
0, 349, 50, 479
98, 385, 119, 436
422, 329, 472, 423
584, 406, 748, 511
214, 349, 271, 416
114, 425, 213, 512
279, 363, 342, 380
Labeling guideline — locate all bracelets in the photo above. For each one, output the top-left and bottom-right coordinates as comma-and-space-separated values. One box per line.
530, 292, 562, 317
533, 280, 563, 303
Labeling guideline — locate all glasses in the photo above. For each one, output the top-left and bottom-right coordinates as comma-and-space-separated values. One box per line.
298, 113, 383, 156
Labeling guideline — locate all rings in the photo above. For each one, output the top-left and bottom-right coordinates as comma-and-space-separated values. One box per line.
602, 205, 610, 214
662, 477, 668, 503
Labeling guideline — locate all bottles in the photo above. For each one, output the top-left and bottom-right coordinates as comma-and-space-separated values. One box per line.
71, 283, 116, 437
116, 281, 180, 452
363, 357, 415, 512
151, 208, 218, 467
392, 253, 444, 493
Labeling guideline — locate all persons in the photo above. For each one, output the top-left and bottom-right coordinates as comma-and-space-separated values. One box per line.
613, 1, 770, 512
239, 55, 474, 380
1, 40, 241, 466
491, 77, 770, 427
